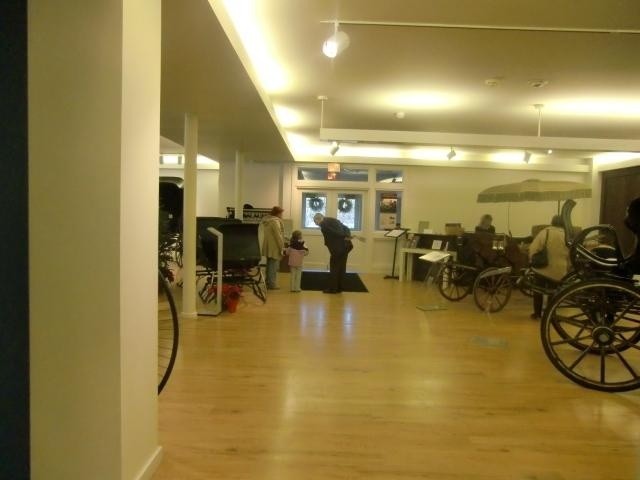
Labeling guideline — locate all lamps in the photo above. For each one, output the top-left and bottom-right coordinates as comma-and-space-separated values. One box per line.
322, 20, 349, 58
524, 149, 531, 162
329, 141, 340, 155
446, 145, 457, 160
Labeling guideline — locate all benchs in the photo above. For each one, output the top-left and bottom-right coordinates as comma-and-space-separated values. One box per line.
560, 198, 639, 278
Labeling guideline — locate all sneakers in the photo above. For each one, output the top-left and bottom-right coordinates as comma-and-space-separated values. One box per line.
529, 312, 542, 321
266, 285, 302, 294
323, 288, 341, 294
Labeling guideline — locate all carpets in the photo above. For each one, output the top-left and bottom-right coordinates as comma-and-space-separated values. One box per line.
300, 271, 368, 292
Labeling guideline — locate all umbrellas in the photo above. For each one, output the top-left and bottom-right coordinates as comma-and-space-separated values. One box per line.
478, 177, 594, 229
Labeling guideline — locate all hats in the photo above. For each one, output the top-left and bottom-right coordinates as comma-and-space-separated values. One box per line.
271, 206, 284, 218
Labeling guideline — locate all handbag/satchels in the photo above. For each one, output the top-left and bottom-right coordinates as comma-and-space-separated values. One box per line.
531, 248, 549, 268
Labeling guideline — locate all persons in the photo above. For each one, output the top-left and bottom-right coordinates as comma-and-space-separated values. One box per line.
527, 215, 573, 322
314, 213, 356, 294
475, 213, 499, 235
286, 230, 310, 292
261, 206, 289, 289
